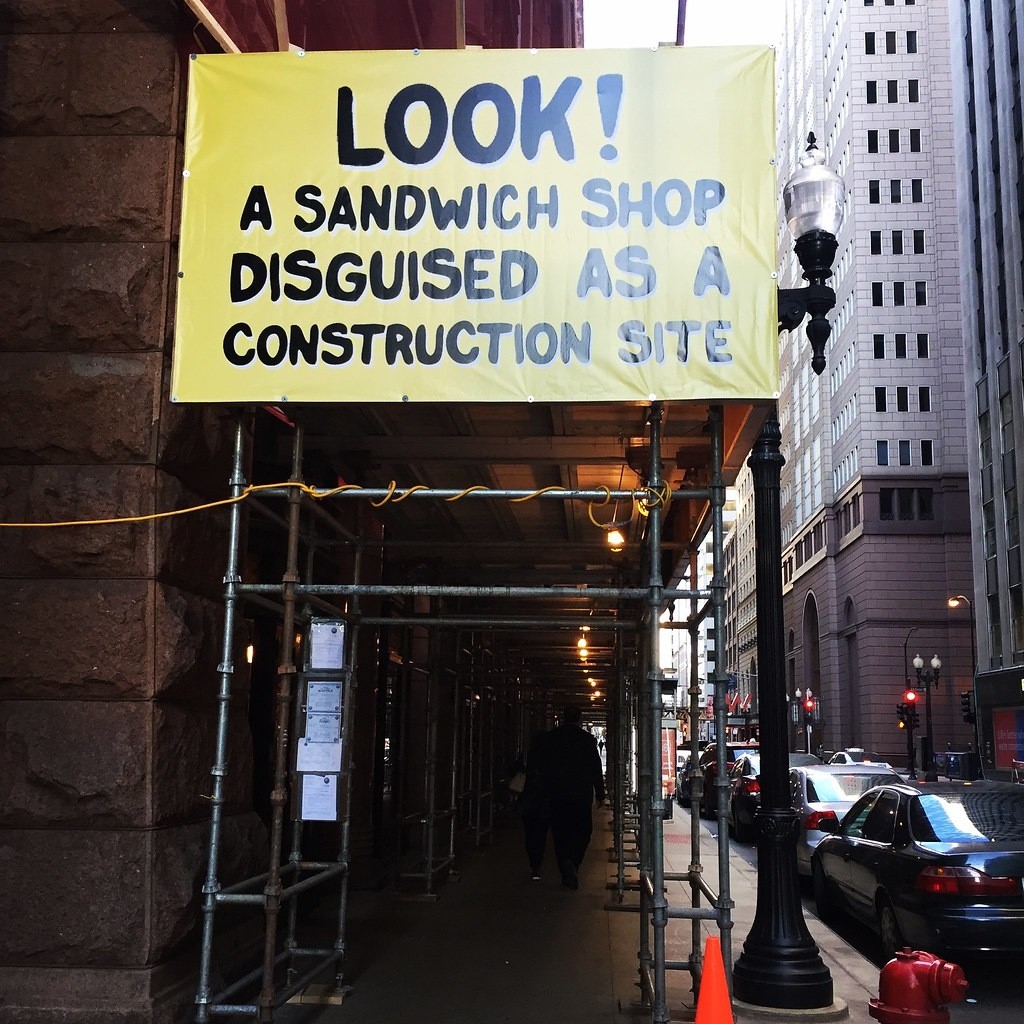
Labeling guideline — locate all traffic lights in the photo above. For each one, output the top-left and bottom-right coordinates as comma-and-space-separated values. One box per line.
896, 703, 908, 721
806, 700, 813, 723
960, 691, 977, 724
897, 721, 906, 729
905, 690, 917, 702
911, 713, 920, 729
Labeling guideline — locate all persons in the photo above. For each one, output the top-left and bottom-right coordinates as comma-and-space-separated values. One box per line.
513, 729, 550, 881
538, 706, 607, 890
599, 740, 604, 751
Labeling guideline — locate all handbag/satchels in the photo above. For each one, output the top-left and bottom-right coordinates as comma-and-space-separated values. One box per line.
508, 752, 527, 793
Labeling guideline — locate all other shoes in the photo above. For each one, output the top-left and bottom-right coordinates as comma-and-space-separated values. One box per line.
532, 872, 545, 881
561, 879, 578, 889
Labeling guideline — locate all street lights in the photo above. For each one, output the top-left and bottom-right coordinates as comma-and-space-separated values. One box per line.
913, 653, 943, 785
794, 687, 814, 754
948, 596, 979, 778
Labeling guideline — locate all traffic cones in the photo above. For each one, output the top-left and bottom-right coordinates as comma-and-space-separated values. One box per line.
693, 937, 738, 1024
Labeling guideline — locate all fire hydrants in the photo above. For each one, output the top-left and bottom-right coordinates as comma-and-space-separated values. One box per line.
868, 948, 969, 1024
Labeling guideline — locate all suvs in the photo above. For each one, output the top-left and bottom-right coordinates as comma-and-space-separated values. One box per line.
699, 738, 759, 819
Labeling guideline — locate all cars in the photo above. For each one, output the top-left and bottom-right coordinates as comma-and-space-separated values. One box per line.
807, 779, 1024, 986
827, 748, 895, 775
727, 753, 825, 842
677, 754, 703, 808
788, 763, 907, 878
